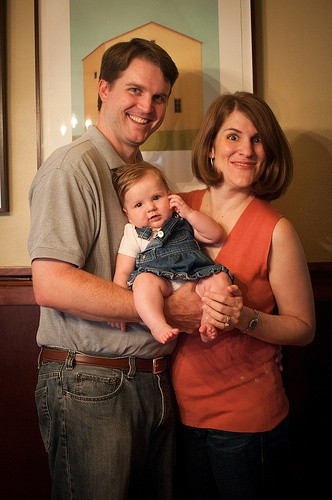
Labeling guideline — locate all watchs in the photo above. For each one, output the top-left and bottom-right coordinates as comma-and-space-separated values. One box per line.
242, 309, 261, 335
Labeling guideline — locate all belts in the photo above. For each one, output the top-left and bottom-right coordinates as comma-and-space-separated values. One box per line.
40, 347, 168, 375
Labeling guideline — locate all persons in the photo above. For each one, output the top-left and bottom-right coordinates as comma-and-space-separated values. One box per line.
27, 37, 206, 500
103, 161, 236, 346
167, 92, 317, 500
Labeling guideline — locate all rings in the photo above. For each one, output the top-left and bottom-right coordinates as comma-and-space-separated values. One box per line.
226, 314, 231, 324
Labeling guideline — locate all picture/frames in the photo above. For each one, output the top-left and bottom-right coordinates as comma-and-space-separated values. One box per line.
36, 0, 269, 193
1, 0, 46, 274
